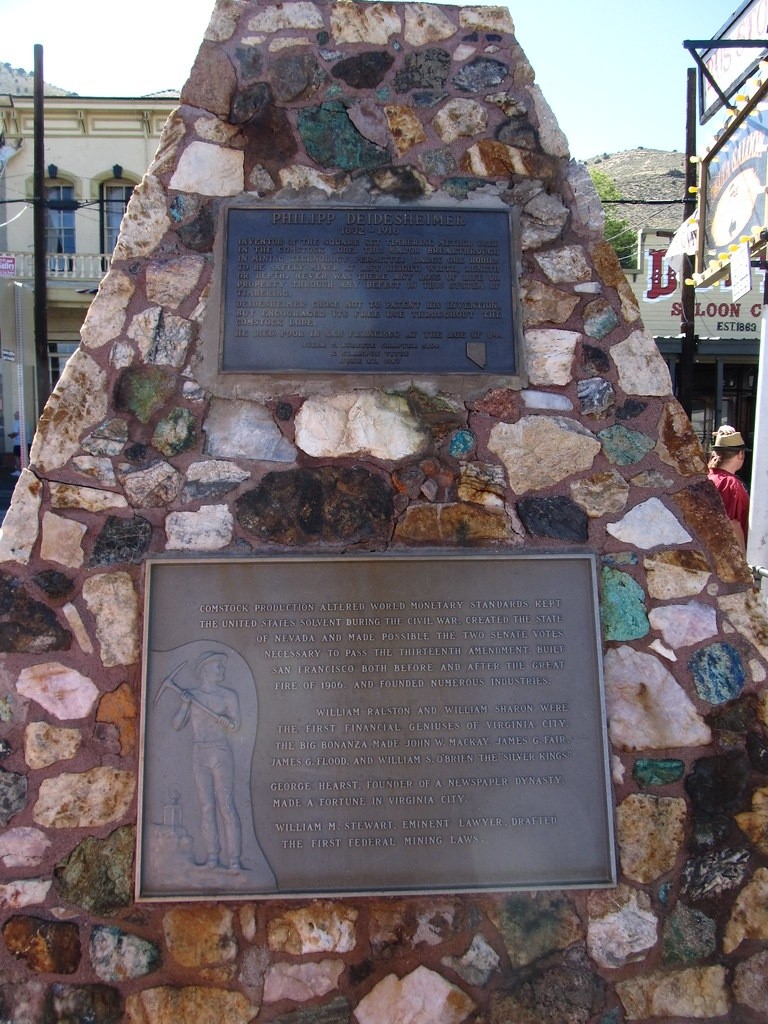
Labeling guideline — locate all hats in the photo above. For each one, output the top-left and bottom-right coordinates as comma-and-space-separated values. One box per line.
712, 432, 752, 452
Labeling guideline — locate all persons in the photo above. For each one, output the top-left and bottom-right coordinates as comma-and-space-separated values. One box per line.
706, 426, 749, 553
